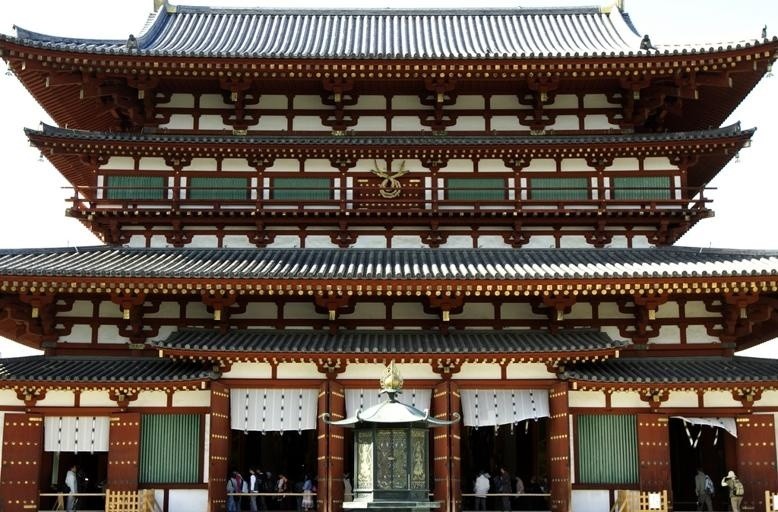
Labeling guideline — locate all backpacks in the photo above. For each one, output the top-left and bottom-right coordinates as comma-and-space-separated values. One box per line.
733, 480, 744, 496
706, 476, 715, 495
283, 478, 291, 491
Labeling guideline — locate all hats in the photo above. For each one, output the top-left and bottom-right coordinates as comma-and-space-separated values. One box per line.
726, 471, 738, 478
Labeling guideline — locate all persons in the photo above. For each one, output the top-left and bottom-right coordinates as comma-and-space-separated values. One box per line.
693, 465, 714, 512
721, 470, 742, 511
65, 465, 78, 512
469, 465, 550, 511
225, 466, 317, 511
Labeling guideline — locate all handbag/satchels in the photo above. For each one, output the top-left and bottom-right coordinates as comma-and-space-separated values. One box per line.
312, 486, 317, 492
